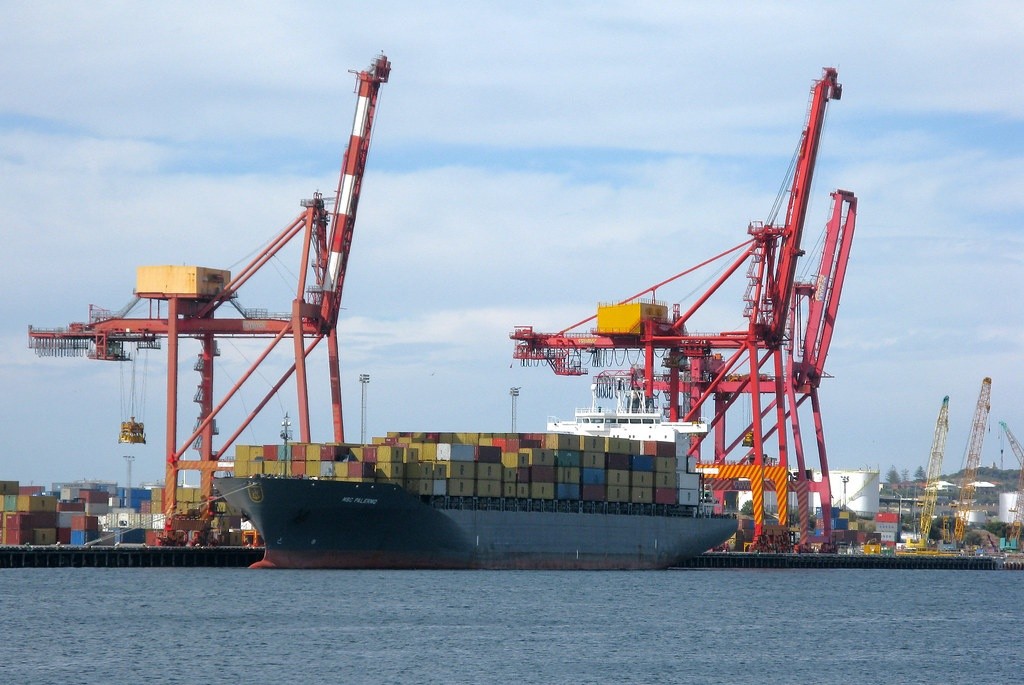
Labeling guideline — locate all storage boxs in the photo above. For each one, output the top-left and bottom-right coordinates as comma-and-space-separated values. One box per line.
232, 430, 700, 507
0, 480, 264, 548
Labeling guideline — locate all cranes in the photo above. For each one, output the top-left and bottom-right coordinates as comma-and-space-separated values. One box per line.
953, 377, 993, 544
507, 62, 841, 553
620, 186, 859, 553
26, 49, 395, 549
999, 416, 1024, 469
906, 395, 950, 548
1005, 453, 1024, 545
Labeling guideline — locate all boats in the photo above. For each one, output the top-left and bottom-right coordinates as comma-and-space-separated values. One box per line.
206, 379, 743, 569
